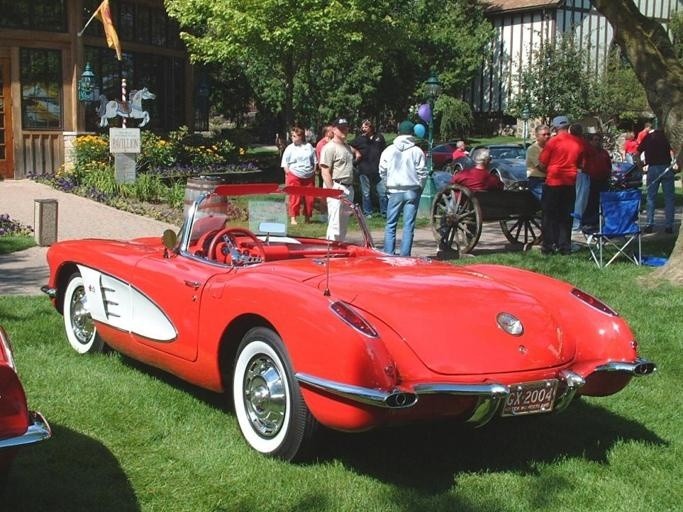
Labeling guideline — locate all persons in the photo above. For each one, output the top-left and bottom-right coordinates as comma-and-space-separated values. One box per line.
623, 122, 674, 234
525, 115, 611, 256
281, 118, 428, 257
449, 141, 499, 244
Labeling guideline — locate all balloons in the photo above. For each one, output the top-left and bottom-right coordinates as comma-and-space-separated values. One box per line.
417, 104, 432, 123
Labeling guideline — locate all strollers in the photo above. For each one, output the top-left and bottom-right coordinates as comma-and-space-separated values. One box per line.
609, 159, 642, 188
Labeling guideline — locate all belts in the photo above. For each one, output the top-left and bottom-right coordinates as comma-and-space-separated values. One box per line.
335, 180, 353, 188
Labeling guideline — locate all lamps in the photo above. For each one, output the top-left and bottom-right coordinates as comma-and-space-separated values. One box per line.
78, 62, 95, 102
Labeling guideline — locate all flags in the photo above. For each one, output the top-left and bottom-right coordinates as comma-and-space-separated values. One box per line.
93, 0, 123, 64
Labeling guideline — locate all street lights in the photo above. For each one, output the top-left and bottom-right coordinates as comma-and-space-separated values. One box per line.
414, 72, 443, 217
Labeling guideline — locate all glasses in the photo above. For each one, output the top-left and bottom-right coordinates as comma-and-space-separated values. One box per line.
540, 133, 550, 136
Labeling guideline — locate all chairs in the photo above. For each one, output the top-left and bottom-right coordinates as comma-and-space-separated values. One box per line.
580, 187, 645, 271
196, 235, 290, 266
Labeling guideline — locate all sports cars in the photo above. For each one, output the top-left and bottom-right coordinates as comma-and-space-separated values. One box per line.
453, 145, 528, 184
43, 184, 658, 462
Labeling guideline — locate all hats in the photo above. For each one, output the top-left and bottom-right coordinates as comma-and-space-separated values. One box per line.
552, 116, 571, 131
333, 116, 352, 130
399, 121, 415, 134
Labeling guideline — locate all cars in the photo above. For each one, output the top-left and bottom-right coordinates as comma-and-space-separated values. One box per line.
0, 321, 53, 451
424, 141, 469, 174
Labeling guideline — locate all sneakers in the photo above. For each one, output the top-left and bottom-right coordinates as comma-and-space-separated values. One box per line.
664, 227, 672, 233
644, 224, 653, 234
